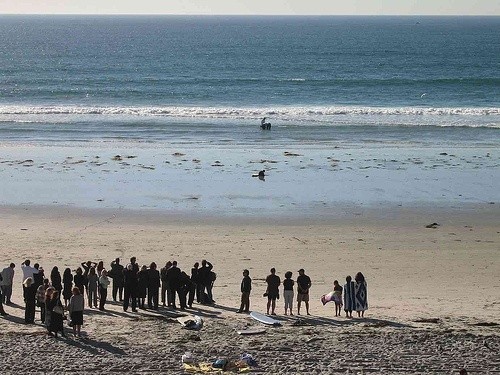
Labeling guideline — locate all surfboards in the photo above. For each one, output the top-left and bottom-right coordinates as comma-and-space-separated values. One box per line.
237, 329, 266, 334
250, 312, 280, 324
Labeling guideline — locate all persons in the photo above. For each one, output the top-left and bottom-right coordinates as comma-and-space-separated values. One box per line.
334, 280, 343, 317
236, 269, 251, 313
261, 118, 271, 130
0, 257, 216, 338
259, 170, 266, 176
354, 272, 368, 317
283, 271, 294, 315
343, 275, 355, 318
265, 268, 280, 315
297, 269, 312, 315
